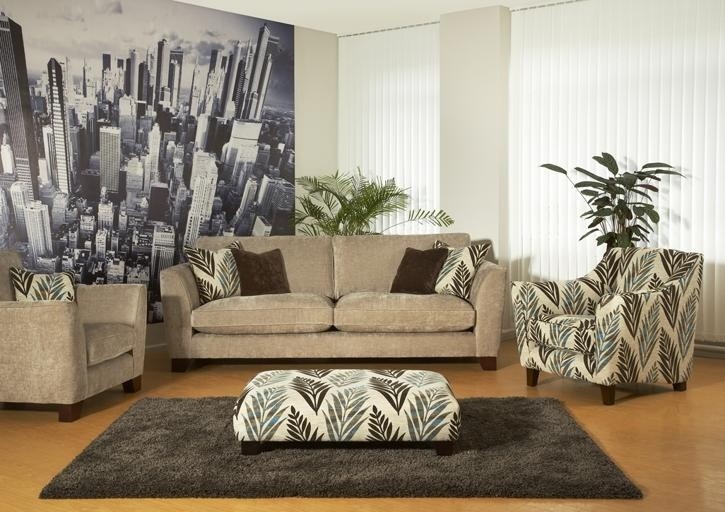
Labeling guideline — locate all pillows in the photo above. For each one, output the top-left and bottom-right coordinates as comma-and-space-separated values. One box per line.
8, 263, 77, 303
389, 239, 493, 300
182, 238, 292, 305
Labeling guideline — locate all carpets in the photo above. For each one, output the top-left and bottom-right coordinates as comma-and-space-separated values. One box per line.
37, 395, 644, 501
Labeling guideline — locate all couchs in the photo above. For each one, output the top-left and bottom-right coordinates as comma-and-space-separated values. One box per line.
159, 232, 508, 372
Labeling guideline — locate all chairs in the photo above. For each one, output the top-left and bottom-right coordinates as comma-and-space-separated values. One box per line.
0, 250, 150, 423
510, 244, 705, 408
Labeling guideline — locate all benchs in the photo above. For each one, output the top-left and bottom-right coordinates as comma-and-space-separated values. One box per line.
231, 368, 463, 458
